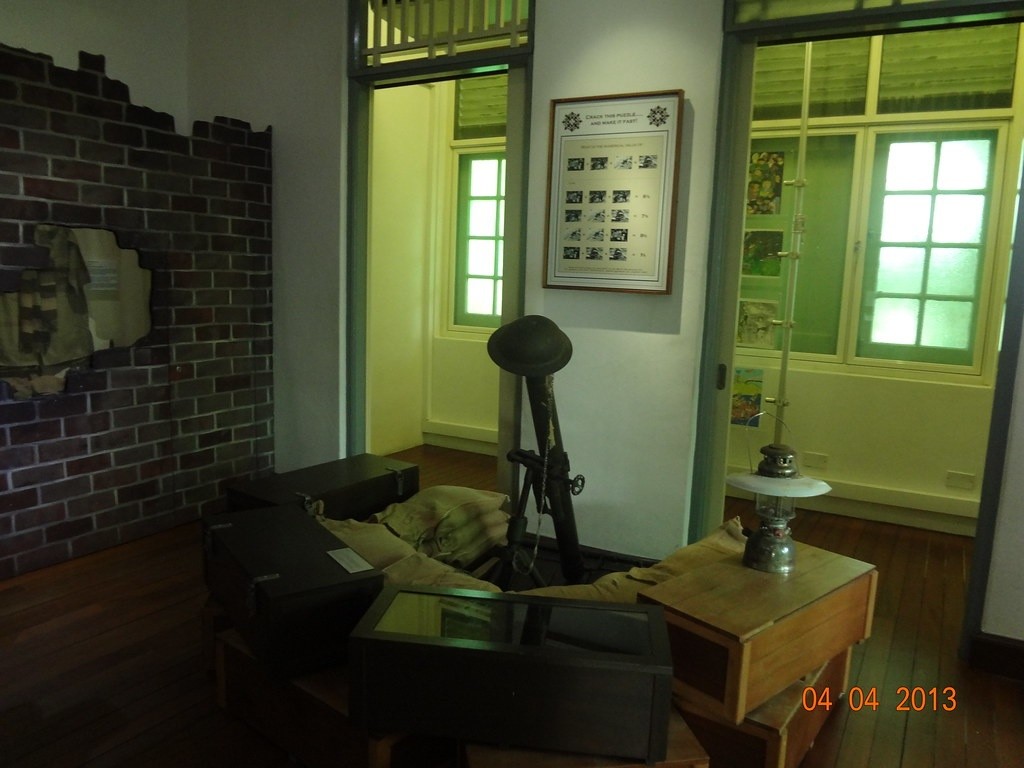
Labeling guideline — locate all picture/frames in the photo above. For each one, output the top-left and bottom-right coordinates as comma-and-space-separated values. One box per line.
540, 89, 685, 295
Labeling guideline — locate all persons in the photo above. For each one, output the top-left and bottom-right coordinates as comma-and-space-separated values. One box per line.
746, 153, 784, 214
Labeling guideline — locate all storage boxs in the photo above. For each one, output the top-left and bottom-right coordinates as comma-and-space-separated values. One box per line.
456, 702, 710, 768
201, 503, 387, 682
224, 453, 421, 523
637, 540, 879, 725
213, 627, 417, 768
672, 645, 852, 768
349, 582, 674, 768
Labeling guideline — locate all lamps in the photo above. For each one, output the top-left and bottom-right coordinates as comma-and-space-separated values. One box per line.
724, 411, 832, 575
